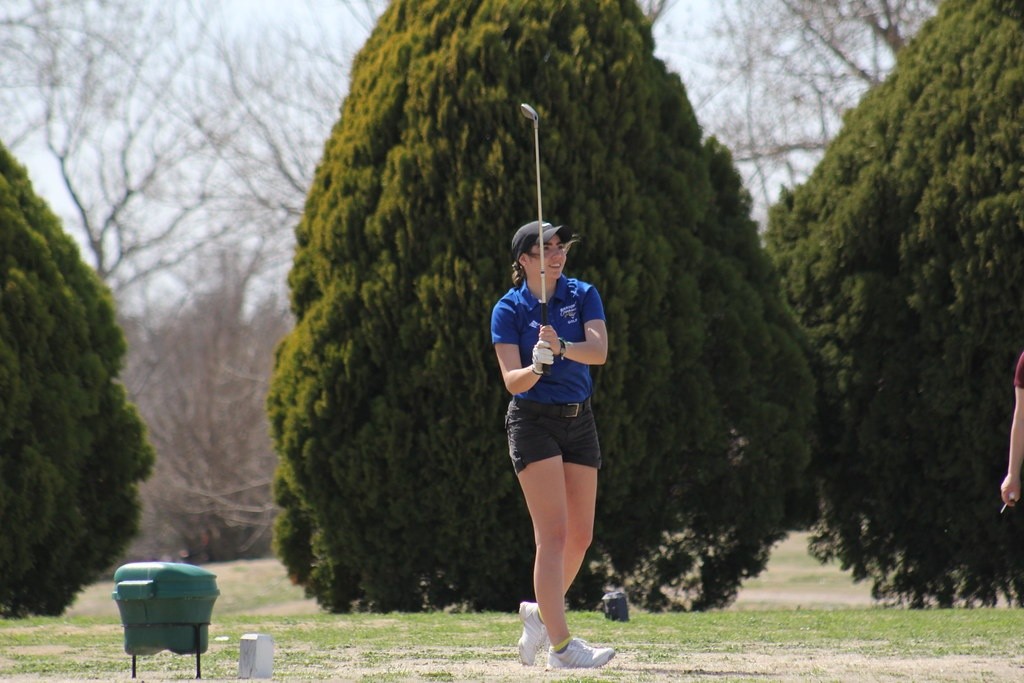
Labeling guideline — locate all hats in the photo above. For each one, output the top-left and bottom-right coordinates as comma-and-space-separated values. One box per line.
511, 221, 573, 266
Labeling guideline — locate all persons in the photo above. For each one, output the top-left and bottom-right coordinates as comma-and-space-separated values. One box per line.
491, 218, 618, 673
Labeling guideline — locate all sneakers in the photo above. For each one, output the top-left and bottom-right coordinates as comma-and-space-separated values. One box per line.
518, 601, 547, 666
547, 637, 615, 669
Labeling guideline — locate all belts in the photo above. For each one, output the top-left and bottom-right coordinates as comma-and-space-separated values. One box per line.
511, 396, 593, 418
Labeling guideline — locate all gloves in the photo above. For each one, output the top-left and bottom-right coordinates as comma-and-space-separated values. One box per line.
532, 340, 554, 375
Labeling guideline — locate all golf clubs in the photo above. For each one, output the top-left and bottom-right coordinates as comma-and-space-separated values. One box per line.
520, 101, 550, 374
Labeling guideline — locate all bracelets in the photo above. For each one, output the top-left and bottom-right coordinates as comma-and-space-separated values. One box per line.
553, 337, 566, 360
